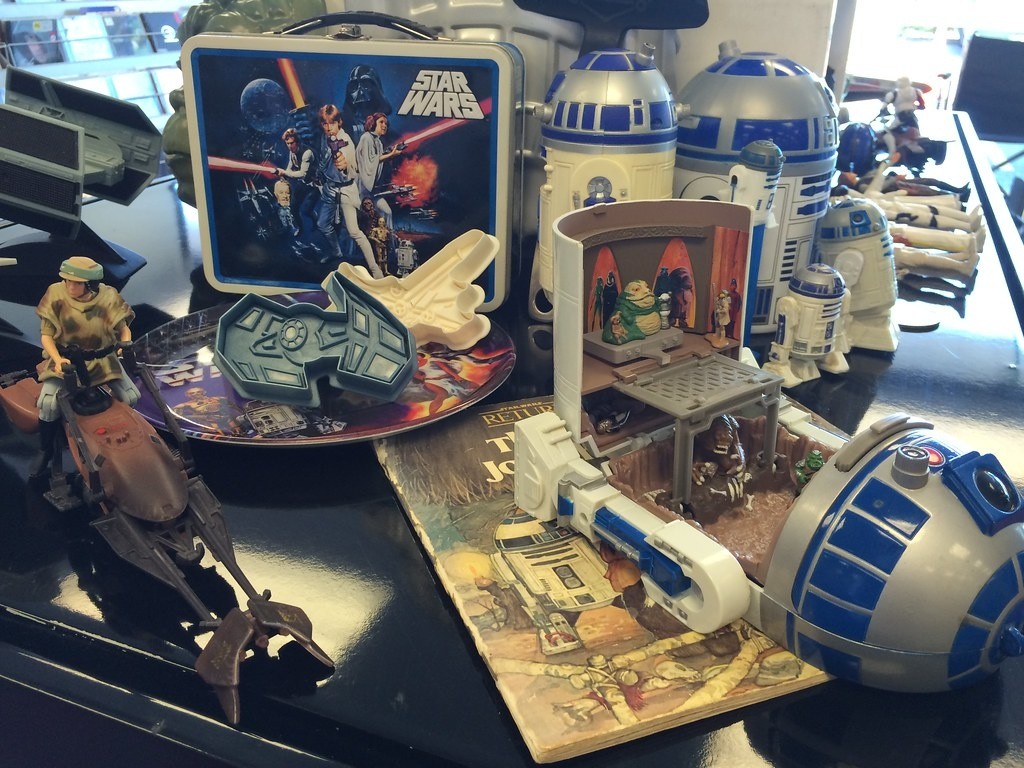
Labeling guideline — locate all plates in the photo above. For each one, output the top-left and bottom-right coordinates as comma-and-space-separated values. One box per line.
118, 291, 516, 446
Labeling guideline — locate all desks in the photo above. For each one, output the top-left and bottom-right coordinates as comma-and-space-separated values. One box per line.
0, 109, 1024, 768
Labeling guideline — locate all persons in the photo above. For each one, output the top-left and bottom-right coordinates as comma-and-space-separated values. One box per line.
28, 256, 144, 480
355, 111, 402, 249
277, 128, 320, 256
314, 103, 384, 279
162, 0, 346, 208
882, 78, 918, 158
713, 289, 731, 339
829, 153, 986, 278
